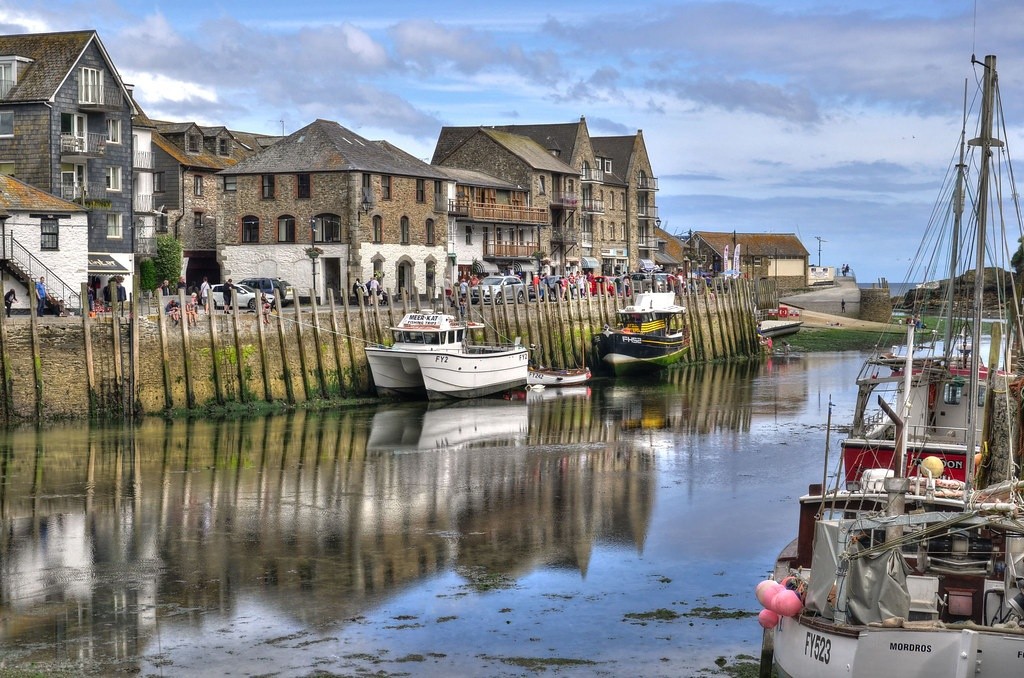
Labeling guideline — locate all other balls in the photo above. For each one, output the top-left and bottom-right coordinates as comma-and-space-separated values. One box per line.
921, 456, 944, 478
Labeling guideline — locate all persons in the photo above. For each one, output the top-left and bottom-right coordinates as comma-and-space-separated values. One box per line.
842, 264, 849, 276
261, 293, 270, 324
4, 289, 18, 317
757, 323, 772, 352
668, 269, 714, 296
201, 277, 211, 314
221, 279, 233, 314
841, 299, 845, 313
87, 282, 95, 312
540, 264, 629, 301
161, 280, 170, 296
352, 277, 379, 306
35, 277, 51, 317
444, 275, 480, 316
177, 277, 186, 294
165, 300, 197, 326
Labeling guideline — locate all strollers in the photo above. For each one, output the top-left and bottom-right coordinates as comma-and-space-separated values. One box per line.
379, 289, 388, 305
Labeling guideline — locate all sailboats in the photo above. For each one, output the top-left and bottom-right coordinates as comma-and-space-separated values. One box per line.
759, 55, 1024, 678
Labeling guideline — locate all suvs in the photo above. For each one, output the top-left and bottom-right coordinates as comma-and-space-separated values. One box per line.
470, 275, 526, 303
529, 275, 571, 301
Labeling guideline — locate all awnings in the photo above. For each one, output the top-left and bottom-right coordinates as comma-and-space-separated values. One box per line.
514, 261, 535, 272
473, 260, 498, 273
581, 257, 600, 268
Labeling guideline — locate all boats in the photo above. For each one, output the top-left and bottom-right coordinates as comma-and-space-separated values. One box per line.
594, 293, 692, 377
364, 310, 529, 400
759, 319, 804, 340
526, 338, 592, 385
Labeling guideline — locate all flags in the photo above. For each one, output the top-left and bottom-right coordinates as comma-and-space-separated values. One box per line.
724, 244, 740, 280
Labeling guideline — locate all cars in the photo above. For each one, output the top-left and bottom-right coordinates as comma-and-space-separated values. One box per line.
591, 273, 674, 296
211, 278, 294, 309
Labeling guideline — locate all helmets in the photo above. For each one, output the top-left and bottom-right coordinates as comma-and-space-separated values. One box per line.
920, 456, 944, 478
